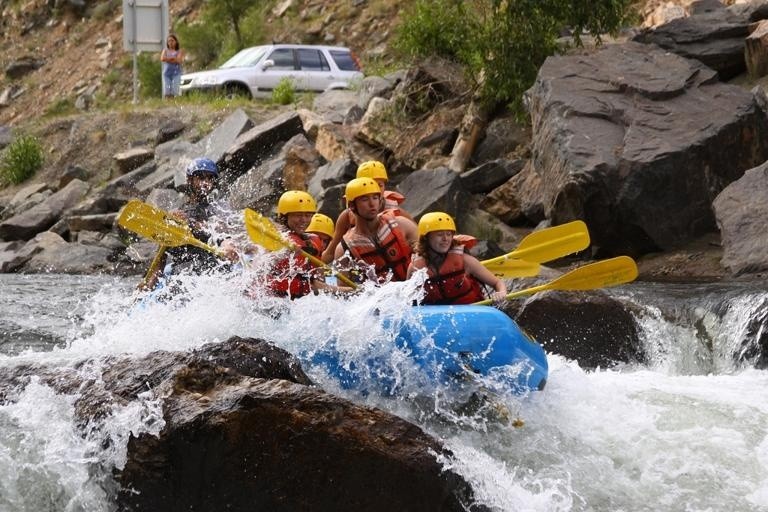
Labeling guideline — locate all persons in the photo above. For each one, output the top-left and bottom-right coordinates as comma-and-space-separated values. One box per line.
158, 158, 242, 275
161, 32, 185, 100
332, 178, 421, 301
303, 211, 334, 258
320, 159, 415, 266
241, 190, 357, 321
403, 211, 508, 309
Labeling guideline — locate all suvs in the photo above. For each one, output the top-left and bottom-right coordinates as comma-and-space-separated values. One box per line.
180, 45, 364, 100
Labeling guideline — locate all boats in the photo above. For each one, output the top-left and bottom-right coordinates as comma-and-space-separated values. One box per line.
140, 286, 547, 396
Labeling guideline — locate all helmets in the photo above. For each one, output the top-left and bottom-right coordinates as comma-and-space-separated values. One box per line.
277, 161, 456, 241
186, 158, 218, 178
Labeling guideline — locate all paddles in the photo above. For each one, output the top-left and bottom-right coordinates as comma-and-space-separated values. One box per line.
482, 219, 592, 266
241, 205, 356, 288
475, 256, 640, 308
494, 265, 545, 279
115, 196, 226, 258
